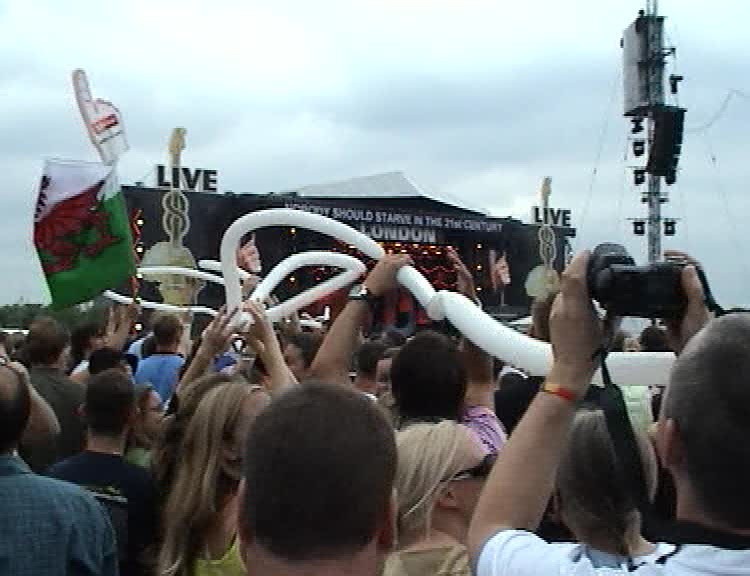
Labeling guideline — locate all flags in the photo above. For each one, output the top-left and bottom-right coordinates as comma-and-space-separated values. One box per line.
34, 161, 137, 311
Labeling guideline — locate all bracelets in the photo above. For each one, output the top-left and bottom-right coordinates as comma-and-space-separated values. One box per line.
538, 383, 581, 411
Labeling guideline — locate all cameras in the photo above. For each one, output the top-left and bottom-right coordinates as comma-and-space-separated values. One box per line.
587, 242, 700, 319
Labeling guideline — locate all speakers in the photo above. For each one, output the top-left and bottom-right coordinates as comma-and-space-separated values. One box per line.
646, 109, 686, 175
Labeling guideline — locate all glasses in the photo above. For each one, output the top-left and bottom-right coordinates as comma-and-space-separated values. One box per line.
442, 452, 498, 484
0, 356, 8, 366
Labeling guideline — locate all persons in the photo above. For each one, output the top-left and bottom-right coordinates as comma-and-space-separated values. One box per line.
0, 251, 750, 576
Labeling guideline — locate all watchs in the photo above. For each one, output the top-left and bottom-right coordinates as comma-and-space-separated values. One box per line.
349, 285, 376, 310
